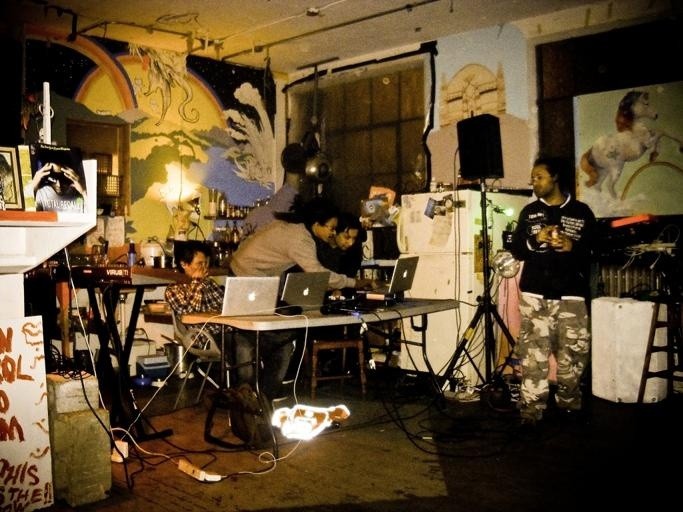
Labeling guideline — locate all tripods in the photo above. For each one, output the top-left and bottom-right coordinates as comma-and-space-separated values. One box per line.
442, 182, 521, 389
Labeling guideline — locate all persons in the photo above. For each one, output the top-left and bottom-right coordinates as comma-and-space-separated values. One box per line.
31, 156, 89, 217
227, 203, 370, 388
166, 243, 258, 391
317, 212, 363, 298
510, 154, 597, 439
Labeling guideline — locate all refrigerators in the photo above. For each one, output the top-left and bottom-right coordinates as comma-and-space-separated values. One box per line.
395, 189, 529, 387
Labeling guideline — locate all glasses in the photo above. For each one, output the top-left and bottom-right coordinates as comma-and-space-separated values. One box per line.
529, 175, 551, 181
186, 256, 211, 269
323, 224, 336, 232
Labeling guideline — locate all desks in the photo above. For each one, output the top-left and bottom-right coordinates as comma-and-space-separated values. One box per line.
188, 298, 460, 448
69, 272, 177, 446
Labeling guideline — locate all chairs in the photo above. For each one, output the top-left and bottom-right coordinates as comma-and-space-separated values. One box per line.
171, 313, 220, 408
309, 337, 367, 399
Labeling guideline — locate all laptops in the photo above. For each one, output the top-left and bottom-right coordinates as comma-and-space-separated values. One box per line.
281, 271, 331, 311
211, 276, 280, 317
356, 256, 419, 296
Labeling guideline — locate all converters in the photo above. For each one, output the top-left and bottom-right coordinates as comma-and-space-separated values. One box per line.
205, 470, 222, 481
449, 379, 458, 392
114, 439, 129, 458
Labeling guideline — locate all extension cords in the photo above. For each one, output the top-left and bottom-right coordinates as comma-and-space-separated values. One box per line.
178, 458, 205, 482
443, 389, 475, 400
111, 453, 125, 463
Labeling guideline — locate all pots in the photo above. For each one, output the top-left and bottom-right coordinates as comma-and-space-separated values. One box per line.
152, 256, 173, 269
280, 131, 332, 183
163, 342, 193, 376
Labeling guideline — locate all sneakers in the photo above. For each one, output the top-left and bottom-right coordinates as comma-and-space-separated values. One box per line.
264, 383, 292, 401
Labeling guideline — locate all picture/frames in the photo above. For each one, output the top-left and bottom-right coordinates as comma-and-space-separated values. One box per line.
0, 142, 25, 211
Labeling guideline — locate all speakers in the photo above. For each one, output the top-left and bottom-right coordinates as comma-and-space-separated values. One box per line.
456, 114, 504, 181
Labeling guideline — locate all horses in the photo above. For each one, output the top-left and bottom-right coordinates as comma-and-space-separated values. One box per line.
580, 90, 682, 199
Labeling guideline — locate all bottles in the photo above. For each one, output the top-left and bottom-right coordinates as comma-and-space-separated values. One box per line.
210, 221, 241, 268
127, 240, 137, 267
207, 188, 272, 219
